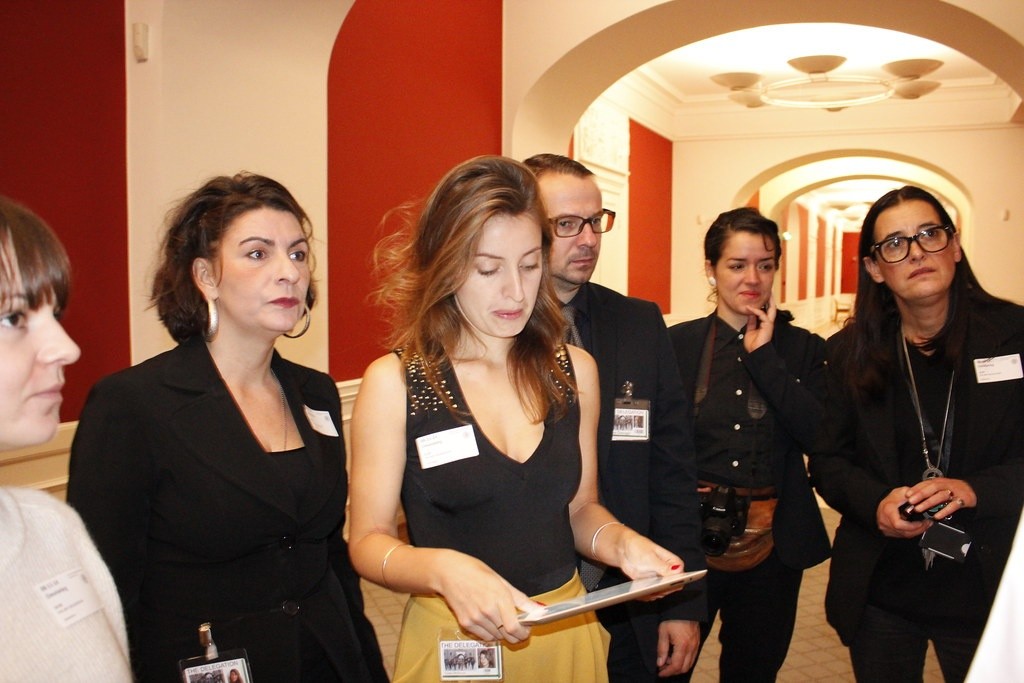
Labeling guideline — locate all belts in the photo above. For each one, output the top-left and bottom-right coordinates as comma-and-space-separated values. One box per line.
697, 479, 778, 497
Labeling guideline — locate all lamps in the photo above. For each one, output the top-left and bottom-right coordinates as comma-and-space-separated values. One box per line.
710, 52, 943, 111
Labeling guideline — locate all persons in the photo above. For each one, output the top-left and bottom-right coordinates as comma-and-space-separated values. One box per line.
614, 419, 627, 430
634, 417, 643, 428
667, 207, 832, 683
523, 153, 707, 683
0, 197, 133, 683
230, 669, 243, 683
66, 175, 389, 683
808, 185, 1024, 683
479, 650, 495, 668
445, 656, 475, 671
349, 154, 684, 683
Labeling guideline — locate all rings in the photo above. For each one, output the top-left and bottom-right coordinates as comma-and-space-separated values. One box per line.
947, 489, 965, 508
497, 625, 504, 629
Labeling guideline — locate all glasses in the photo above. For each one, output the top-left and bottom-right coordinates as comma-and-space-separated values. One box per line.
548, 208, 616, 238
871, 224, 954, 263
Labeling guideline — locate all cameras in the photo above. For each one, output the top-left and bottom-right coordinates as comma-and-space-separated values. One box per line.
698, 484, 750, 556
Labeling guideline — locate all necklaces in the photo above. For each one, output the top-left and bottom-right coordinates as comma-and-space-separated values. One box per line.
901, 322, 955, 481
271, 368, 287, 451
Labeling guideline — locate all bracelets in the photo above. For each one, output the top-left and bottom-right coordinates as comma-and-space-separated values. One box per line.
591, 522, 624, 561
382, 544, 413, 588
